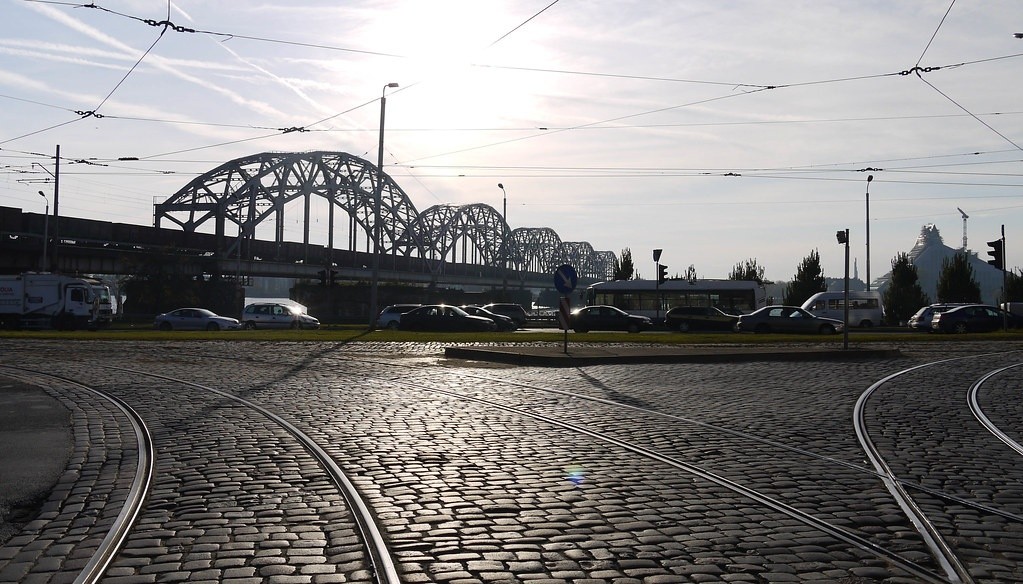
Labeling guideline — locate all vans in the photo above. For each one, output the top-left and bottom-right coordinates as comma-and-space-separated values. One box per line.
789, 290, 883, 331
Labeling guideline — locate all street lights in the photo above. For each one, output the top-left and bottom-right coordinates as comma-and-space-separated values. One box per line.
39, 191, 49, 272
369, 83, 400, 332
498, 183, 507, 303
836, 229, 850, 350
866, 175, 873, 309
31, 145, 60, 275
653, 250, 663, 331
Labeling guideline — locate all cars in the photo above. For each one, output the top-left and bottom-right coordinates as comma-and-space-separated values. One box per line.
569, 306, 653, 334
663, 305, 844, 334
154, 301, 320, 332
377, 302, 531, 333
907, 303, 1018, 334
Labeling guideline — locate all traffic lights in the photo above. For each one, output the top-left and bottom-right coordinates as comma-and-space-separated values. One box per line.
659, 265, 668, 285
986, 239, 1003, 271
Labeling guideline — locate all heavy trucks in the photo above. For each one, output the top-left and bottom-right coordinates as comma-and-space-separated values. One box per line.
0, 274, 113, 330
